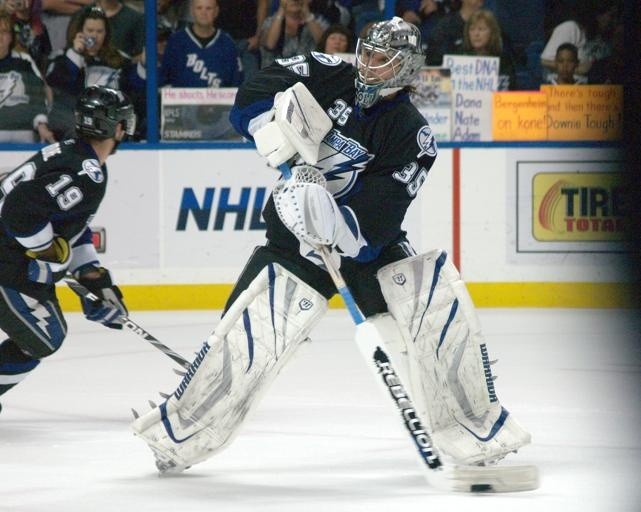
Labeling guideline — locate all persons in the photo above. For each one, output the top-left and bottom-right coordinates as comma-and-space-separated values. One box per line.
1, 0, 641, 145
1, 86, 128, 399
132, 16, 536, 497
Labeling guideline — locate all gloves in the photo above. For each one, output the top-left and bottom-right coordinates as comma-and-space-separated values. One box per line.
72, 260, 129, 328
25, 234, 72, 296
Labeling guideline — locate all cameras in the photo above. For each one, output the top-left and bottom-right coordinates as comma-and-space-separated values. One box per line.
80, 35, 96, 50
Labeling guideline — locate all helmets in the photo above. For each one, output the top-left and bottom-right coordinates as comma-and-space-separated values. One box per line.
74, 85, 138, 142
353, 17, 426, 112
